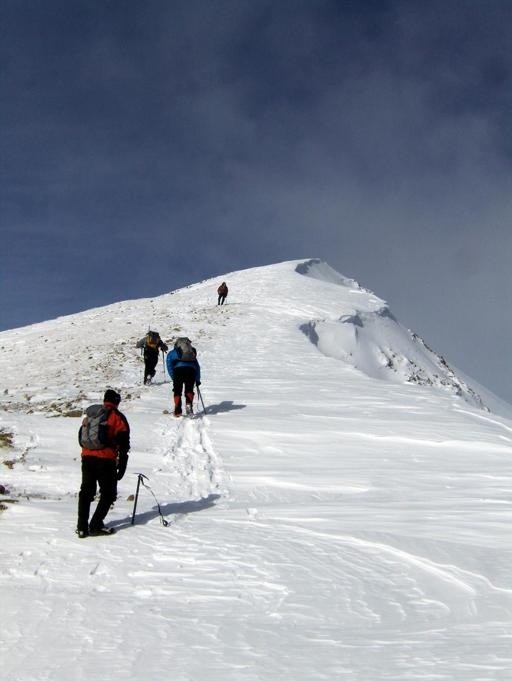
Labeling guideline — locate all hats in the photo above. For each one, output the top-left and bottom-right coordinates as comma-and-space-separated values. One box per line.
104, 389, 121, 404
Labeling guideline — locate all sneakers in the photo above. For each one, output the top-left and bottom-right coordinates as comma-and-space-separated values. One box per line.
77, 525, 115, 538
173, 407, 182, 417
144, 377, 151, 385
184, 404, 194, 417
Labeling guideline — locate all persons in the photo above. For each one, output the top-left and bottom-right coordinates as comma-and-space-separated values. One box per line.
135, 330, 167, 386
75, 389, 131, 538
216, 282, 229, 306
165, 337, 202, 420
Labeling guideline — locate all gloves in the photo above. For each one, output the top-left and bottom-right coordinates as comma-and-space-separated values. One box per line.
116, 462, 126, 480
195, 380, 201, 387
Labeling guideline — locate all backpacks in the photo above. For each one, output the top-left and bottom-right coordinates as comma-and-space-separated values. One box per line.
174, 336, 197, 360
78, 404, 110, 450
218, 285, 225, 296
146, 331, 160, 348
